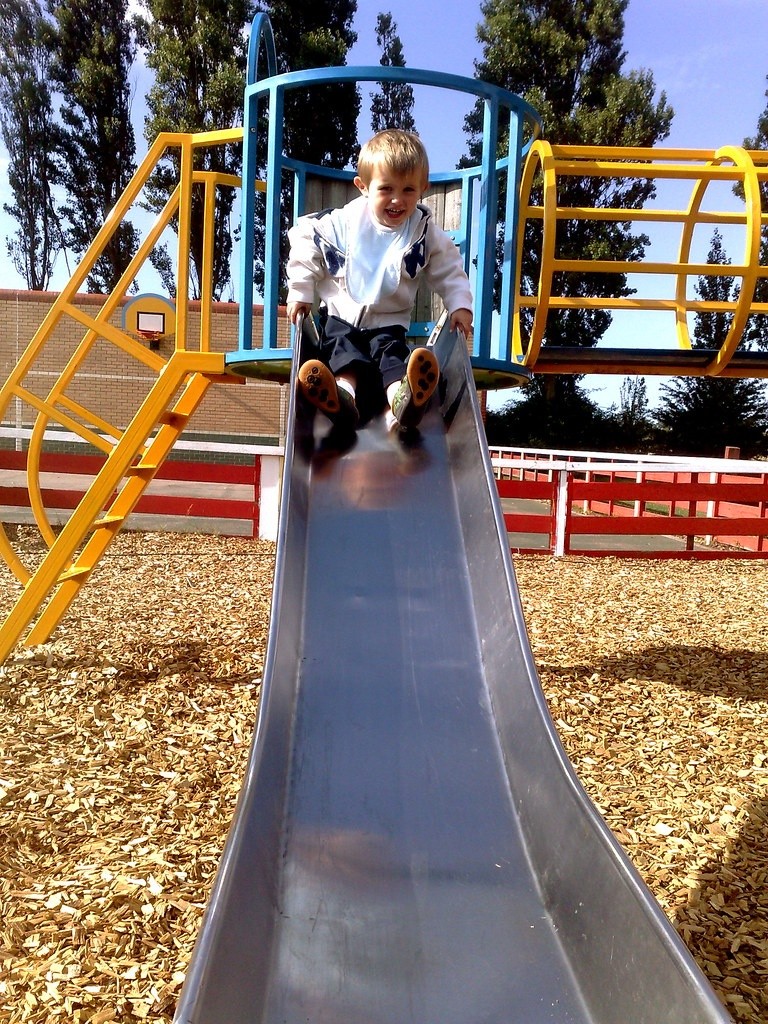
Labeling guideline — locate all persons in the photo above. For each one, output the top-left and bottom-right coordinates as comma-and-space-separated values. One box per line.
286, 129, 473, 431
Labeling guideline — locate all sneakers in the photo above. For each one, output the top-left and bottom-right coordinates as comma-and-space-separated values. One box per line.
298, 358, 360, 434
391, 347, 440, 428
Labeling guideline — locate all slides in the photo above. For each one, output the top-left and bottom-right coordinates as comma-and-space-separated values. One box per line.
171, 310, 744, 1024
